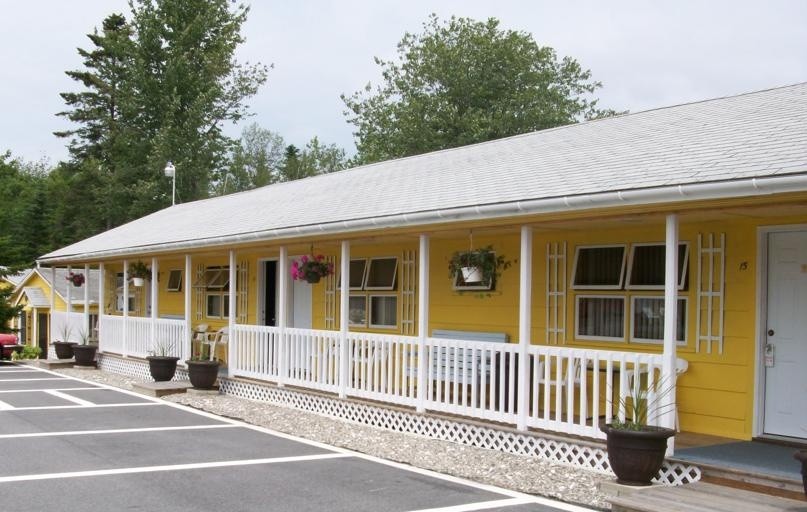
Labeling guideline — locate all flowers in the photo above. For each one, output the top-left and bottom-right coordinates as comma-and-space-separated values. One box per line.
66, 271, 86, 287
290, 254, 334, 283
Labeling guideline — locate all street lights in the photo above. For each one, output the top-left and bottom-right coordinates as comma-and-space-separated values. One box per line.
164, 162, 176, 205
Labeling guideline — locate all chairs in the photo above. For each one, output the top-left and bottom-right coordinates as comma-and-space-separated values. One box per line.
191, 323, 233, 363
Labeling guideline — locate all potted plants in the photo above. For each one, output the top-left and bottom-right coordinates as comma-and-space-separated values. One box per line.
121, 258, 156, 287
186, 335, 225, 390
51, 321, 101, 366
149, 337, 180, 382
443, 245, 517, 290
596, 353, 685, 487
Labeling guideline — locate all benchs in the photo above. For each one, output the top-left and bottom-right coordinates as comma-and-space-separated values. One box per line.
404, 330, 509, 404
155, 313, 185, 328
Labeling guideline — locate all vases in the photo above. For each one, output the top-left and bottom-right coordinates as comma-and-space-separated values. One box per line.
306, 272, 320, 284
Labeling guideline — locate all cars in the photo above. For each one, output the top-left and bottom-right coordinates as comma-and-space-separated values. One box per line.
0, 334, 24, 360
